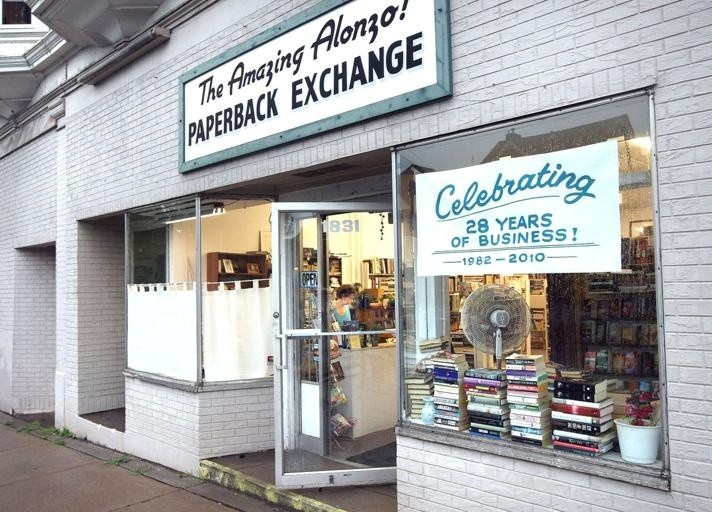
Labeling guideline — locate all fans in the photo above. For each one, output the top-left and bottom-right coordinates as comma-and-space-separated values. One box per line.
460, 283, 532, 373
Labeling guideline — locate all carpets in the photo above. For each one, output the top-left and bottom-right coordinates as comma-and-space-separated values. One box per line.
343, 440, 397, 467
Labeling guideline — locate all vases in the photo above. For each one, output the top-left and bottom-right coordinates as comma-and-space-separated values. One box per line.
612, 416, 660, 465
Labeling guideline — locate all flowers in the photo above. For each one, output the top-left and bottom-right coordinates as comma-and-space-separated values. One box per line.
622, 388, 659, 426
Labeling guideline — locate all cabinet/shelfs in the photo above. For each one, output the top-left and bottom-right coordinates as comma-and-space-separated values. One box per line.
205, 252, 341, 301
447, 274, 548, 368
362, 258, 394, 295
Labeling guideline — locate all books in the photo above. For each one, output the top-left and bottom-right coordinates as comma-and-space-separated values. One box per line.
218, 247, 395, 435
